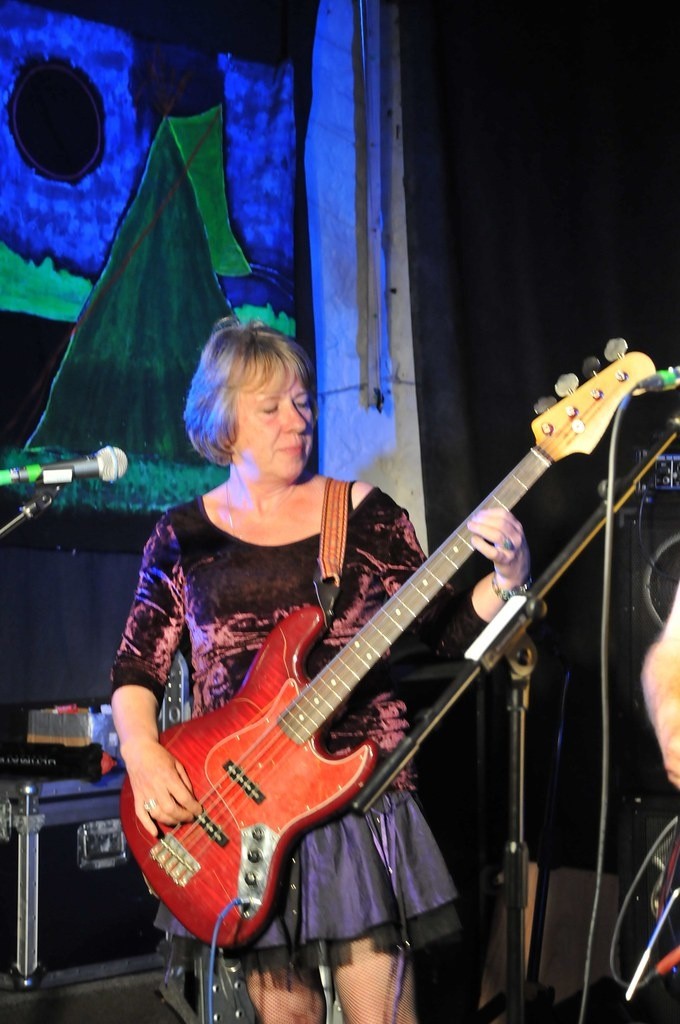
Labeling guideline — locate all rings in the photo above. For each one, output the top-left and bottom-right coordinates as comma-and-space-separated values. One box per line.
144, 800, 156, 810
503, 540, 512, 550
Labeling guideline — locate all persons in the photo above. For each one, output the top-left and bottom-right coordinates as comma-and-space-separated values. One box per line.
109, 327, 531, 1024
637, 579, 679, 787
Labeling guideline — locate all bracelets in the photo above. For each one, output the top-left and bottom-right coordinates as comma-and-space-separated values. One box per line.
492, 577, 532, 601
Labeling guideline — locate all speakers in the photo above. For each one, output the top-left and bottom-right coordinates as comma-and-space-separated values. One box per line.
616, 505, 680, 795
617, 794, 680, 1024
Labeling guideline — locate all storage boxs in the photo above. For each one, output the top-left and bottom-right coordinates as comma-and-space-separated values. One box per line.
28, 703, 118, 749
0, 773, 172, 989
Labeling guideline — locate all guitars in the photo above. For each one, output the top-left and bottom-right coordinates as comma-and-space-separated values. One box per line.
118, 337, 659, 955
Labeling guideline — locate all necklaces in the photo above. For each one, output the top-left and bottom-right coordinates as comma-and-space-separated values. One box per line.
225, 480, 297, 536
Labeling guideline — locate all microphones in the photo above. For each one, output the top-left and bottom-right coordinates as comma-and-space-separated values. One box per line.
0, 447, 128, 486
